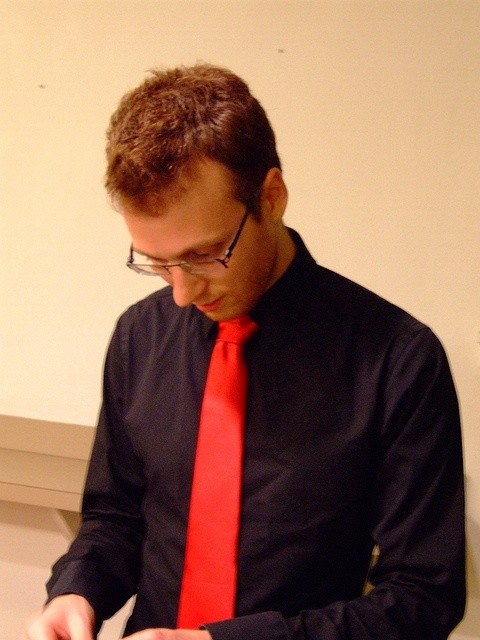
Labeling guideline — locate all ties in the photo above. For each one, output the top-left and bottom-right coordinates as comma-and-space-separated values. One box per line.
176, 315, 258, 631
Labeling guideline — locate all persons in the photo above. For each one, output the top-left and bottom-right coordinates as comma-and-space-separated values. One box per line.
33, 59, 466, 636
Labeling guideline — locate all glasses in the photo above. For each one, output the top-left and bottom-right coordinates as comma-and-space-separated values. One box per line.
125, 208, 251, 275
